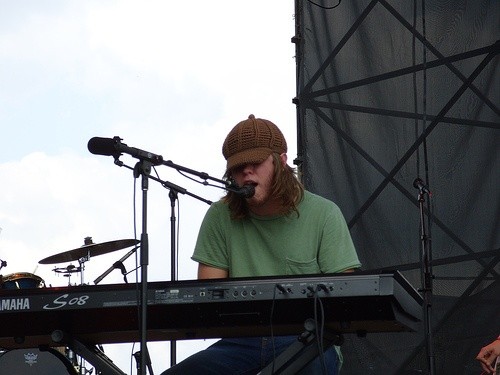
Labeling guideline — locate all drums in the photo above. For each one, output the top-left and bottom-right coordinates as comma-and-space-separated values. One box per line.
1, 273, 46, 289
0, 346, 77, 375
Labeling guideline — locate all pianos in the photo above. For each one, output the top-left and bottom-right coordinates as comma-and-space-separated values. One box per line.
0, 266, 425, 375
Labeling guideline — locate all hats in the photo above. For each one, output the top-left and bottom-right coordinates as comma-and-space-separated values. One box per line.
221, 114, 288, 180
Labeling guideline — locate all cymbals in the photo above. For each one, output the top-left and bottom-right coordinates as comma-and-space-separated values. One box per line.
39, 237, 143, 264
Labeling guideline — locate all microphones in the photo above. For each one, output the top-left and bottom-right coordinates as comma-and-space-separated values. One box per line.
239, 183, 255, 197
93, 246, 138, 284
87, 137, 124, 156
413, 178, 431, 195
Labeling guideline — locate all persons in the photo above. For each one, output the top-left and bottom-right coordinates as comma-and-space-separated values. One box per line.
159, 114, 362, 375
475, 336, 500, 375
